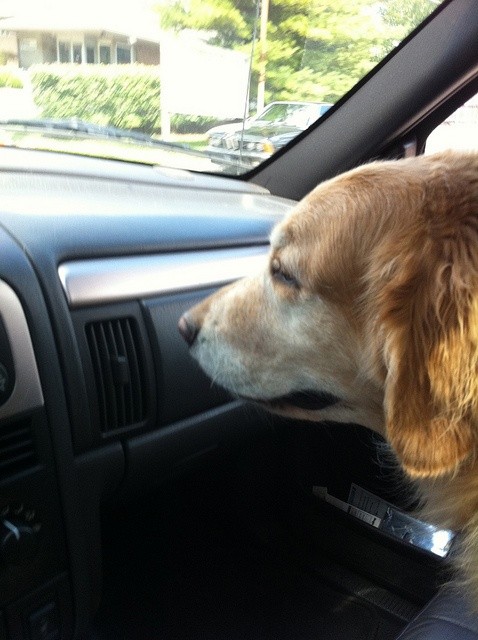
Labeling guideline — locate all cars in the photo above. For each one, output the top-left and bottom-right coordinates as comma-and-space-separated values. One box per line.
207, 101, 334, 171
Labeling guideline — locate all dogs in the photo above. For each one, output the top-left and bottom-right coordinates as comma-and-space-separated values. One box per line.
179, 149, 478, 638
